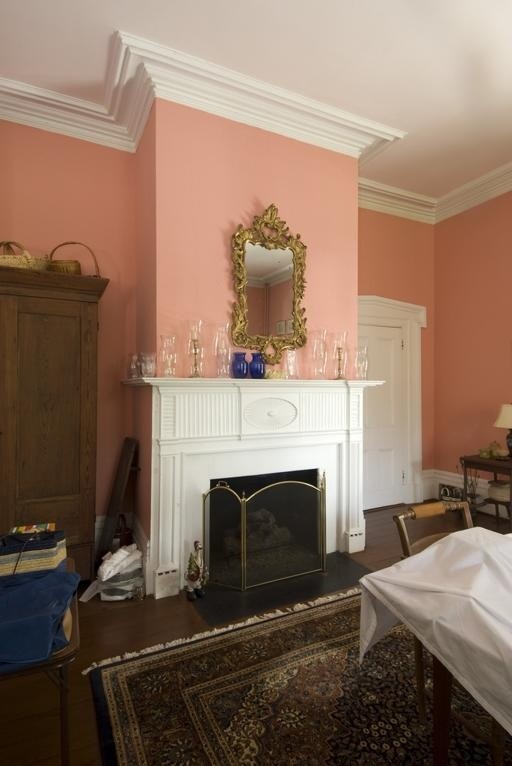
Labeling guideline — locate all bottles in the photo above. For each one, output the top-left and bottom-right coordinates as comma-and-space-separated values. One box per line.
124, 320, 367, 378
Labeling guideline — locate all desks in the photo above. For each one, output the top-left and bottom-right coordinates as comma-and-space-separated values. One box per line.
0, 558, 81, 765
460, 454, 512, 528
357, 527, 510, 765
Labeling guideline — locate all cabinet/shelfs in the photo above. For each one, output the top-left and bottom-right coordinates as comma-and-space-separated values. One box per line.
0, 264, 108, 585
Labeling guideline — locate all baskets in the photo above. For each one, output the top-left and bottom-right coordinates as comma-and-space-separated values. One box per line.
0, 239, 100, 278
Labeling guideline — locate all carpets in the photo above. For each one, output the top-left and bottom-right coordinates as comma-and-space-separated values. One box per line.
82, 585, 511, 765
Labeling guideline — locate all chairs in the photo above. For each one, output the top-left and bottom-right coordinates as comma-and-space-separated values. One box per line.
392, 498, 504, 764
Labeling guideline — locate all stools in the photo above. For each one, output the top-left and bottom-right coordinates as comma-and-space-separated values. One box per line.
483, 481, 510, 528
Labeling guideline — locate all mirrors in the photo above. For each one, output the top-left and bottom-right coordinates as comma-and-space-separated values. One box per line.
232, 204, 306, 362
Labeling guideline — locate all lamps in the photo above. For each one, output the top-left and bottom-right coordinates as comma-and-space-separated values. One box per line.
493, 403, 512, 461
